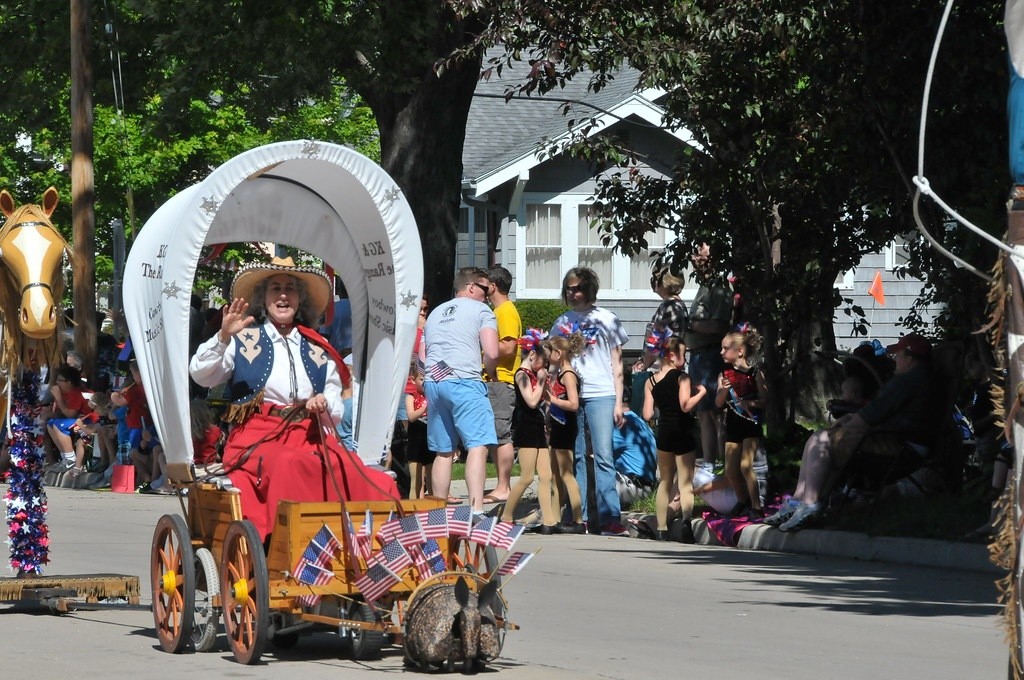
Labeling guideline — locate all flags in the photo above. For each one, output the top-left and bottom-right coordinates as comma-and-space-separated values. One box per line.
429, 360, 452, 382
290, 524, 342, 608
344, 508, 428, 572
413, 353, 425, 374
350, 564, 400, 602
416, 505, 533, 576
408, 537, 445, 582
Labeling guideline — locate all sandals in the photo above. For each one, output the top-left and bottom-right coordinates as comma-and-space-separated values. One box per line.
448, 497, 463, 504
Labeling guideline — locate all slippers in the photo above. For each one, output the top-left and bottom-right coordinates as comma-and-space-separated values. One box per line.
631, 521, 656, 539
482, 493, 508, 504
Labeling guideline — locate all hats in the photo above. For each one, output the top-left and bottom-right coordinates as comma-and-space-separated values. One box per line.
623, 386, 631, 403
230, 255, 332, 328
886, 334, 932, 361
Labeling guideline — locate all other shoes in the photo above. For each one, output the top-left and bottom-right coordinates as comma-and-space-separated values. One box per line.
555, 520, 586, 534
50, 458, 173, 496
682, 519, 695, 543
727, 502, 748, 518
541, 523, 557, 534
751, 508, 764, 523
656, 531, 671, 540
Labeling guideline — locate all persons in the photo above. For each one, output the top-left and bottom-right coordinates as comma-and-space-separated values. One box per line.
45, 361, 178, 493
517, 385, 657, 523
60, 308, 120, 368
764, 328, 1015, 544
339, 347, 353, 450
189, 398, 224, 475
550, 267, 631, 535
386, 260, 521, 516
630, 235, 775, 544
190, 257, 403, 544
496, 322, 586, 532
318, 277, 352, 355
186, 292, 232, 360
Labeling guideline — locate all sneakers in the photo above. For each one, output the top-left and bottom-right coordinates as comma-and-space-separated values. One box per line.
778, 502, 822, 533
763, 500, 799, 525
600, 519, 630, 536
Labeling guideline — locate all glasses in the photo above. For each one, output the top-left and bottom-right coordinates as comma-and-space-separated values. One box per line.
420, 306, 429, 313
466, 282, 490, 295
566, 285, 585, 293
57, 378, 67, 382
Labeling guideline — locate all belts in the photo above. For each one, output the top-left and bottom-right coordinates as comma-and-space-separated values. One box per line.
629, 473, 653, 487
253, 405, 313, 423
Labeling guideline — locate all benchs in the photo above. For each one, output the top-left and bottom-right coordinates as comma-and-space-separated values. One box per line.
164, 462, 396, 490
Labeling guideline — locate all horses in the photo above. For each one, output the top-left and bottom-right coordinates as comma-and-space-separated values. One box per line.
0, 185, 66, 431
